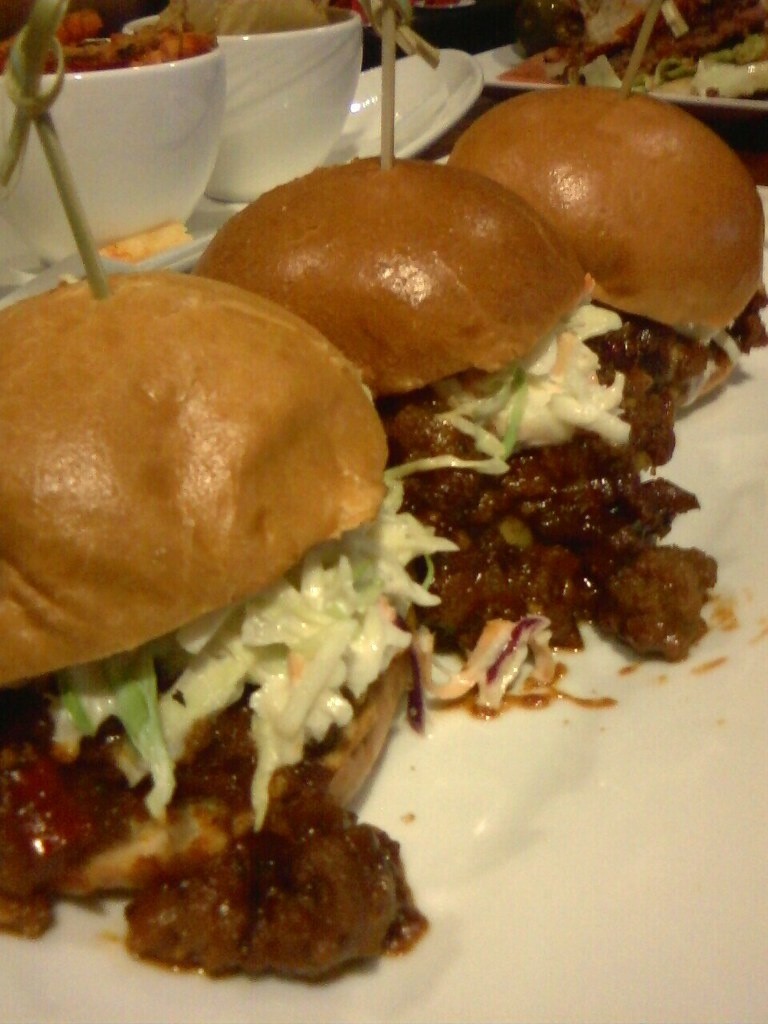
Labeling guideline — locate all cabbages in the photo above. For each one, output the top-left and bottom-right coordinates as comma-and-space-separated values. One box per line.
575, 34, 768, 100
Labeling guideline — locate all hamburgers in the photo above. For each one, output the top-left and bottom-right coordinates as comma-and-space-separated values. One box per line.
0, 87, 768, 980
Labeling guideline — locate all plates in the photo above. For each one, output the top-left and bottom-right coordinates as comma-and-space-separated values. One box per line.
473, 43, 768, 125
0, 48, 485, 313
0, 357, 768, 1024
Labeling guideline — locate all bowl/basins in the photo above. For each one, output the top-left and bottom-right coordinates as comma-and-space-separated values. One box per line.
123, 9, 365, 204
0, 46, 228, 270
